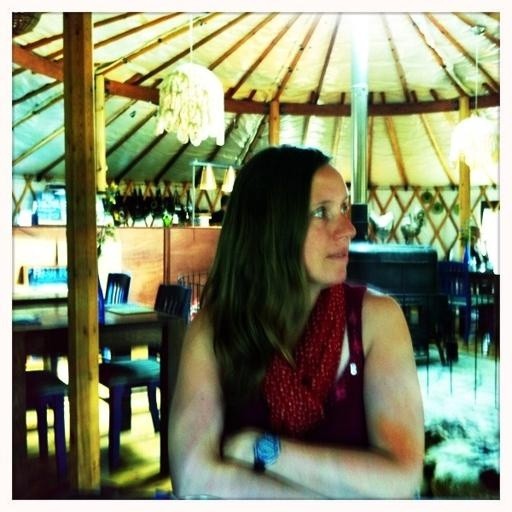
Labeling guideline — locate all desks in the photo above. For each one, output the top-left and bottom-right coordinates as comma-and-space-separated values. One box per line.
13, 299, 188, 481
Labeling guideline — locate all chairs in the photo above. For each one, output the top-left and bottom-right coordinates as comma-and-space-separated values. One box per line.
94, 270, 192, 472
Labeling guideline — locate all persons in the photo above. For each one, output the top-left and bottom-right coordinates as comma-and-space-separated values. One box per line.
167, 145, 425, 499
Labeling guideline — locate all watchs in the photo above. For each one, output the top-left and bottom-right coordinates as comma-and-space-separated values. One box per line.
252, 430, 280, 473
